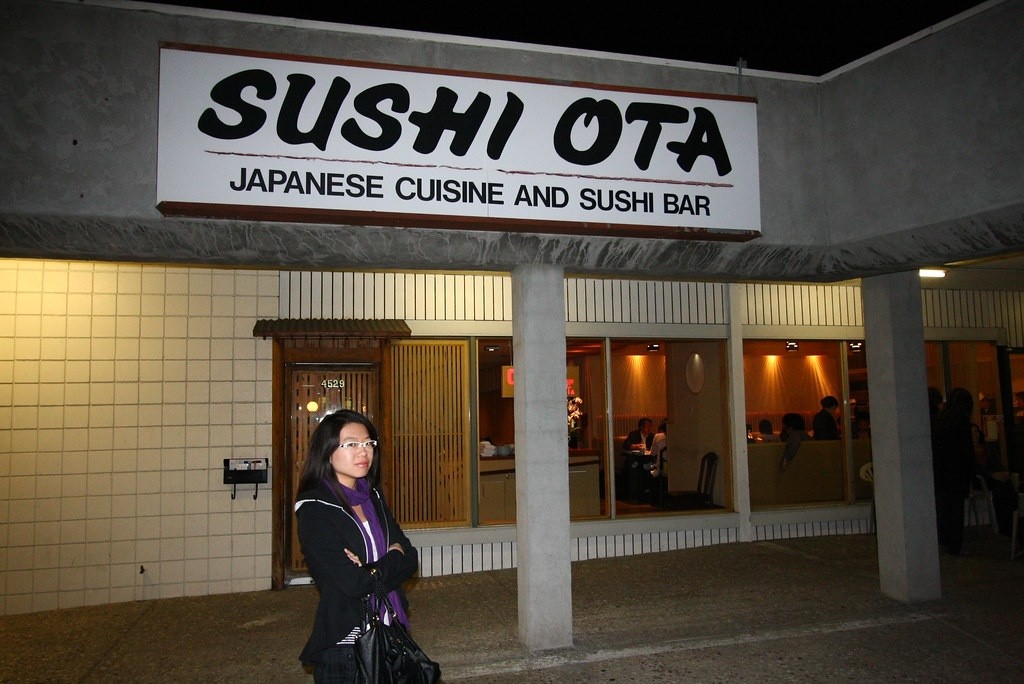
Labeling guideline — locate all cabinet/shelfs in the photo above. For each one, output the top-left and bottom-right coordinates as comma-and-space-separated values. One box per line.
479, 448, 600, 519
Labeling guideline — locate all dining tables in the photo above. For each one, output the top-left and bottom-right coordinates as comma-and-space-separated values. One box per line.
621, 450, 652, 501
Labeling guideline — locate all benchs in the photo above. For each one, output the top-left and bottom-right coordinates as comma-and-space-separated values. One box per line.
748, 440, 874, 505
614, 435, 638, 496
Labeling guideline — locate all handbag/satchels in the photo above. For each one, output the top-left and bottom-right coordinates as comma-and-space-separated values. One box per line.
354, 563, 441, 684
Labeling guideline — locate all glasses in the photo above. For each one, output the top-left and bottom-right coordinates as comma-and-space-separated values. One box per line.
339, 440, 377, 450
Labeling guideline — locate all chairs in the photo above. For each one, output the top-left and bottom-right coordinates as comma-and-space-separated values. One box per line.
666, 452, 718, 509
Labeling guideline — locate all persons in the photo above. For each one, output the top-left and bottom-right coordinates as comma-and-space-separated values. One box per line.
779, 413, 813, 473
812, 396, 841, 441
297, 409, 418, 684
1014, 391, 1024, 416
649, 418, 668, 506
930, 388, 975, 557
624, 418, 654, 451
757, 418, 779, 442
979, 391, 997, 416
852, 409, 871, 439
971, 423, 1019, 537
